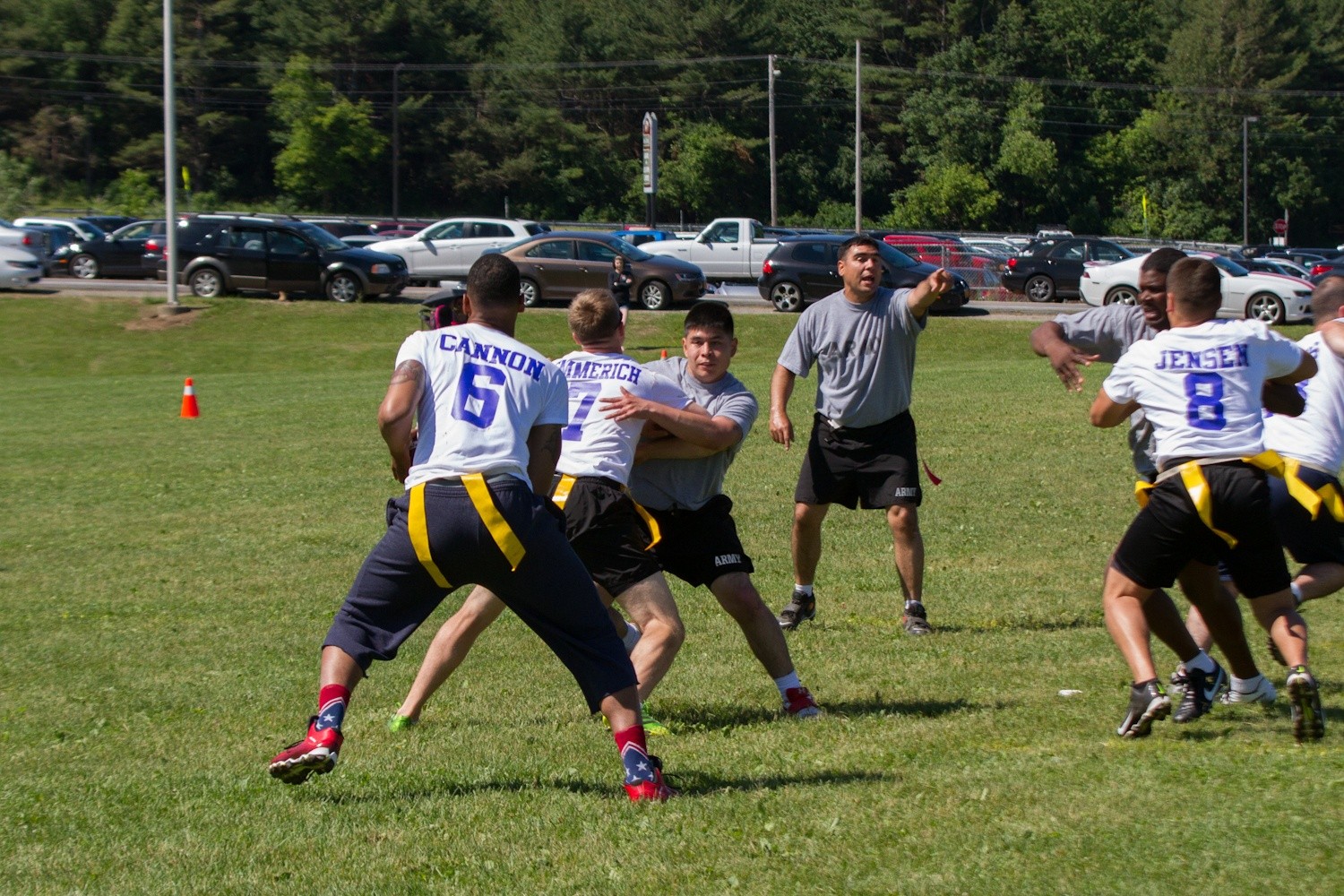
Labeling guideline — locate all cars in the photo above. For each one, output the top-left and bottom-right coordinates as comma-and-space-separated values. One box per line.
495, 229, 706, 312
0, 204, 1343, 331
756, 233, 971, 315
999, 235, 1138, 304
1077, 249, 1320, 326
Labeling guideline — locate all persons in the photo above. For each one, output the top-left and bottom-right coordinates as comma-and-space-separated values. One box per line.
269, 253, 820, 802
1033, 246, 1344, 745
770, 234, 952, 633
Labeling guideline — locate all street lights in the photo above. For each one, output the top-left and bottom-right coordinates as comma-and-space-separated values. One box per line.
1243, 116, 1258, 246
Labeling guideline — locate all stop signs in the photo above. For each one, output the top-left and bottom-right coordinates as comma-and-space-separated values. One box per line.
1273, 218, 1287, 233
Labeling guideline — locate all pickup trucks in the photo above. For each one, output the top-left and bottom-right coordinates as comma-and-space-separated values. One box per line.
634, 215, 801, 285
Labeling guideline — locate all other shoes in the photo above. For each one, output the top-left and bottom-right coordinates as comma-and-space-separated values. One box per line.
1167, 668, 1188, 694
1266, 636, 1288, 666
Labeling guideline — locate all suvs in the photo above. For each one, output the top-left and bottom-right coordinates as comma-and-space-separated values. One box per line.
358, 214, 550, 286
152, 209, 409, 305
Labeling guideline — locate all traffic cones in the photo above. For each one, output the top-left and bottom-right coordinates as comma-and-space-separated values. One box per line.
179, 376, 199, 418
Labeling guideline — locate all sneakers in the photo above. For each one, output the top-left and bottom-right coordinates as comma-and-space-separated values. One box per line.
268, 715, 344, 785
903, 603, 932, 636
1172, 656, 1228, 723
624, 755, 686, 803
782, 687, 822, 720
1117, 678, 1172, 739
1219, 677, 1276, 710
779, 587, 815, 632
602, 707, 678, 740
387, 714, 419, 738
1286, 664, 1325, 747
